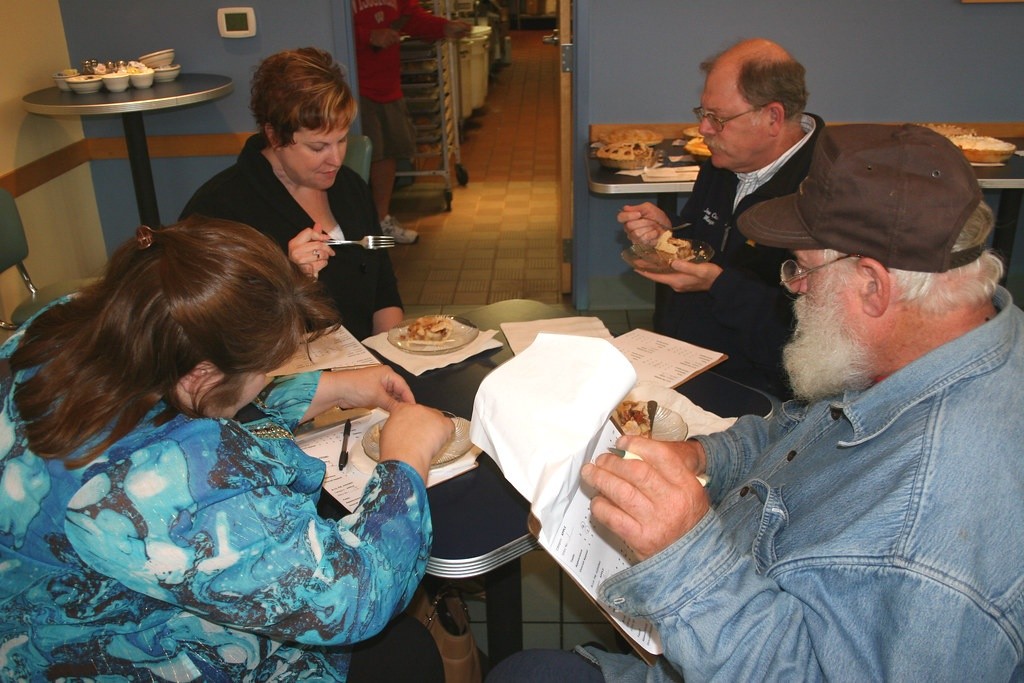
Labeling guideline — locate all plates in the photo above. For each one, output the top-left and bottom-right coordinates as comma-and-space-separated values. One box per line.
961, 146, 1019, 163
612, 401, 690, 443
362, 414, 474, 470
387, 314, 479, 355
621, 237, 715, 273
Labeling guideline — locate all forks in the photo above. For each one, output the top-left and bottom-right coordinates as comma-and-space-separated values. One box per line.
639, 217, 691, 230
322, 235, 395, 250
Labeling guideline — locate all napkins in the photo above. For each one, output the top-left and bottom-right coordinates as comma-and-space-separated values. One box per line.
361, 324, 504, 376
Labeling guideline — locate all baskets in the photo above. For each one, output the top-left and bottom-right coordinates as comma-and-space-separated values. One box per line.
961, 143, 1017, 164
597, 149, 658, 170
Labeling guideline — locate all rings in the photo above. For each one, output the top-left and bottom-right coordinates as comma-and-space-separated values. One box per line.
313, 249, 320, 261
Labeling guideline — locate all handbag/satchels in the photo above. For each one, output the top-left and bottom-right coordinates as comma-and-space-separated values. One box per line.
403, 577, 487, 683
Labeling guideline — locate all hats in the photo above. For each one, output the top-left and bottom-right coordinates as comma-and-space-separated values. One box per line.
736, 123, 986, 273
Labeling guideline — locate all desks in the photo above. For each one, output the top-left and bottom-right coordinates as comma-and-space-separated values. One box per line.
22, 74, 235, 230
293, 293, 776, 672
587, 138, 1024, 327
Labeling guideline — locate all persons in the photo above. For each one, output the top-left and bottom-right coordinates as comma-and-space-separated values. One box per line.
177, 50, 403, 342
0, 218, 447, 683
618, 37, 827, 421
446, 121, 1024, 683
351, 0, 475, 242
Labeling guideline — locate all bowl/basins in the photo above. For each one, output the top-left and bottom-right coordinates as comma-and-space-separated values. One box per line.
154, 65, 181, 83
138, 48, 176, 68
129, 69, 155, 90
65, 74, 103, 95
101, 72, 129, 93
52, 72, 80, 91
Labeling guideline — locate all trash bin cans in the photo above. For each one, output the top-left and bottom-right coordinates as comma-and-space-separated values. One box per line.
459, 40, 475, 116
471, 25, 493, 112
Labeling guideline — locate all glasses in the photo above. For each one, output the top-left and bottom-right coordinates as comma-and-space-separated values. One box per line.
780, 254, 890, 294
692, 100, 786, 132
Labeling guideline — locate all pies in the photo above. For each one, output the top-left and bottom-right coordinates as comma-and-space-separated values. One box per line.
594, 126, 712, 170
915, 124, 1015, 163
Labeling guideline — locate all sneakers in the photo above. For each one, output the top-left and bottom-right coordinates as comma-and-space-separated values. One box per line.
381, 215, 420, 244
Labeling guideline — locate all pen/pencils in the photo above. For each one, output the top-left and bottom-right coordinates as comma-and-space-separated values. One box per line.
338, 419, 351, 470
